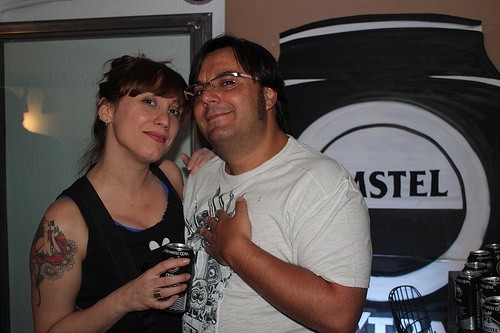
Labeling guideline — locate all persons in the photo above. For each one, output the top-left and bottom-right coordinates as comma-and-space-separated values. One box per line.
30, 56, 217, 333
183, 31, 373, 333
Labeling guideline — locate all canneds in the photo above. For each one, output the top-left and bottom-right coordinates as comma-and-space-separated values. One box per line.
454, 244, 500, 333
162, 243, 195, 311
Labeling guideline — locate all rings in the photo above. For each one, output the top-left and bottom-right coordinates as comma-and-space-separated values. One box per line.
153, 288, 163, 300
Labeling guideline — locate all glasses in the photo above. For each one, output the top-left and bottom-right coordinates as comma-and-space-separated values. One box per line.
184, 71, 260, 100
